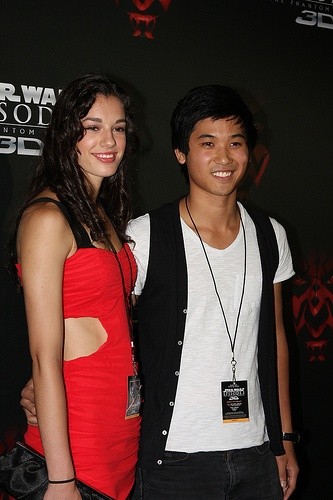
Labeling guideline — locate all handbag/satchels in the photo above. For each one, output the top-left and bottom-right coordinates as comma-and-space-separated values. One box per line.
0, 442, 115, 500
